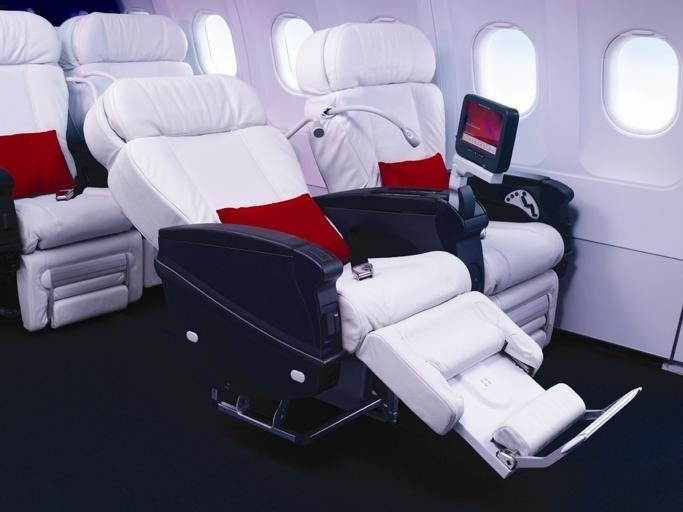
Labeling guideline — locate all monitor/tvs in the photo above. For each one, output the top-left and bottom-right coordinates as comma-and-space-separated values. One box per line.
454, 93, 519, 174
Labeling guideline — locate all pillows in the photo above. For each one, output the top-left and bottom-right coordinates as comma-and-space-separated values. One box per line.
1, 129, 74, 201
216, 194, 352, 266
376, 153, 448, 194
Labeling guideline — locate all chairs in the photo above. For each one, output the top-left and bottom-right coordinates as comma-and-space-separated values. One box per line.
83, 73, 644, 478
0, 9, 144, 331
54, 10, 237, 291
296, 19, 575, 351
